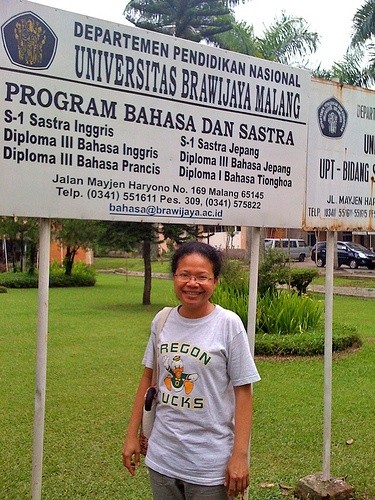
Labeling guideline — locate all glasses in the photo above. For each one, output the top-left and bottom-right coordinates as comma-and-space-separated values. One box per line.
173, 273, 214, 284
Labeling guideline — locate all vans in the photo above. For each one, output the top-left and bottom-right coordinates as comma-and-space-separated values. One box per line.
263, 239, 310, 261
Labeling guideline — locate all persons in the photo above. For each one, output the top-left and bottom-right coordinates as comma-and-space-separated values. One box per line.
120, 240, 263, 499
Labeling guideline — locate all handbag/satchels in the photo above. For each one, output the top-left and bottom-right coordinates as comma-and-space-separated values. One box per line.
138, 386, 158, 456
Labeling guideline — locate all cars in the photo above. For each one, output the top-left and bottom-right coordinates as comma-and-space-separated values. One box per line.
312, 241, 375, 269
